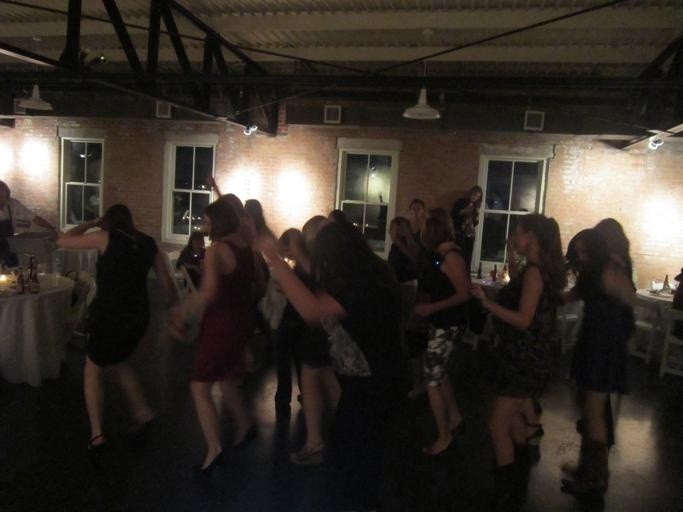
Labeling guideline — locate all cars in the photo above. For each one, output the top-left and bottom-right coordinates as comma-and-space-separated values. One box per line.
648, 280, 656, 291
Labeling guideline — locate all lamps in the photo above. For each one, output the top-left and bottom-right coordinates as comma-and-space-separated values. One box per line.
657, 307, 683, 379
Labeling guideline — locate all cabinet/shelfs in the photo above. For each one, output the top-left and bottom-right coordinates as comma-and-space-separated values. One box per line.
492, 264, 497, 281
477, 262, 483, 280
17, 255, 40, 296
663, 274, 670, 291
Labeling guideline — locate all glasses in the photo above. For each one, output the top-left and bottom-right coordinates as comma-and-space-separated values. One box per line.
401, 59, 440, 121
18, 37, 52, 111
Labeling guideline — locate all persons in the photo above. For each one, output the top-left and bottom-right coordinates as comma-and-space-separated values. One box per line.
0, 181, 56, 268
469, 212, 571, 487
169, 174, 472, 489
55, 203, 172, 451
561, 219, 634, 491
451, 185, 483, 262
671, 270, 682, 338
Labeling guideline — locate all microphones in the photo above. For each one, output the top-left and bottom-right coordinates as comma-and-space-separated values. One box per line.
630, 319, 658, 365
52, 247, 97, 279
